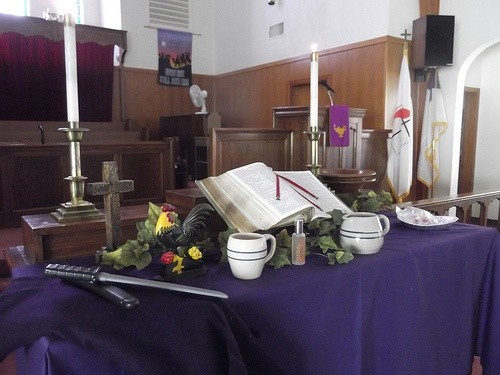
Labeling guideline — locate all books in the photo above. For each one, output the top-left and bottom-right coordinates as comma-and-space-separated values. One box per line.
194, 163, 355, 234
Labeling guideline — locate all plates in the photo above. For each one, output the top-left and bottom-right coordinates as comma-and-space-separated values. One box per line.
396, 215, 459, 230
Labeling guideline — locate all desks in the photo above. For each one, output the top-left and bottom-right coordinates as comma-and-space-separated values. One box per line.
1, 210, 499, 375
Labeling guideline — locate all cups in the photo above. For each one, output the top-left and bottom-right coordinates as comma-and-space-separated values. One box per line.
226, 232, 276, 280
339, 212, 390, 254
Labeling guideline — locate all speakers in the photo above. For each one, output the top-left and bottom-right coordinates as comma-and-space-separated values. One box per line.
410, 14, 455, 69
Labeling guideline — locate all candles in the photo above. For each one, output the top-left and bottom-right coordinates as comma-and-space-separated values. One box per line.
63, 14, 79, 122
309, 44, 318, 126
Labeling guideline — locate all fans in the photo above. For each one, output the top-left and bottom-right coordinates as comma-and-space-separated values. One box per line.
190, 85, 208, 114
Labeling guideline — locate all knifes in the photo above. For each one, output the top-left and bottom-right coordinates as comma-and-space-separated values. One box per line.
45, 263, 228, 298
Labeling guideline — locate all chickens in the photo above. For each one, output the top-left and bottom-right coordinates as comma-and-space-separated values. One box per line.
155, 202, 229, 274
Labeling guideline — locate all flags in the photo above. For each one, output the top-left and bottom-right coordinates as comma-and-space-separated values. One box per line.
386, 56, 413, 204
417, 69, 448, 188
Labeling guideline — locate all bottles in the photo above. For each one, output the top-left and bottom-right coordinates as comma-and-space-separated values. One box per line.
292, 219, 305, 265
